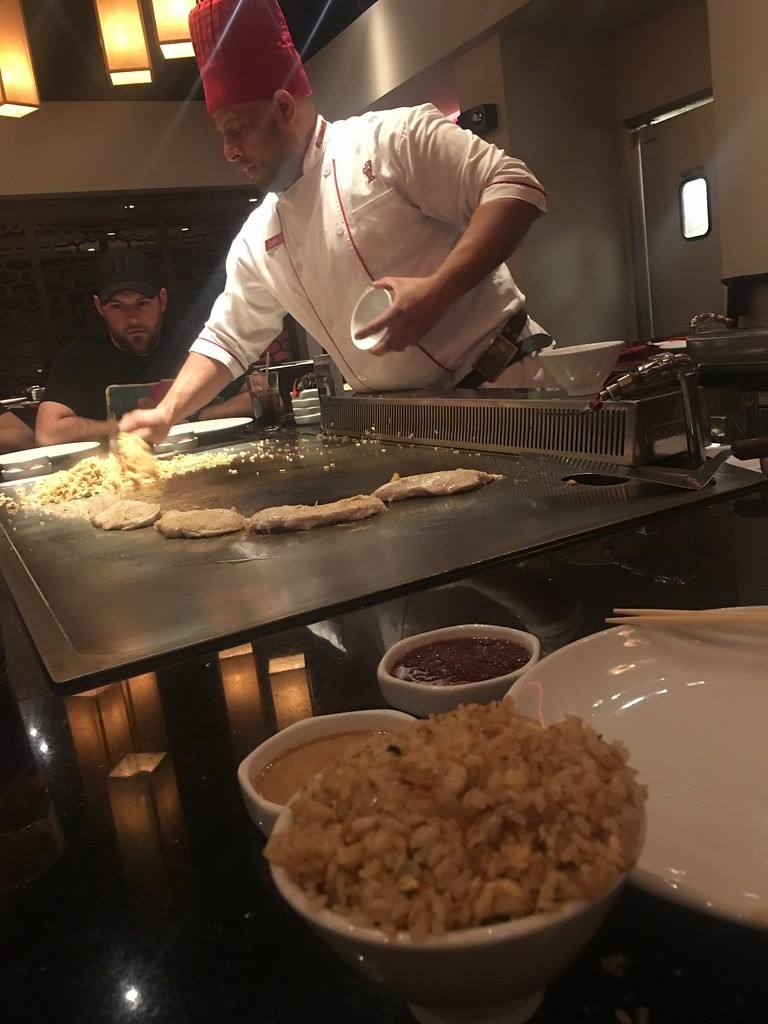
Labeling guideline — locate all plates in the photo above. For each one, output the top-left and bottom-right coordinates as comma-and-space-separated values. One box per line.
501, 605, 768, 928
659, 339, 688, 349
288, 381, 356, 425
0, 441, 102, 466
170, 417, 254, 440
0, 397, 27, 405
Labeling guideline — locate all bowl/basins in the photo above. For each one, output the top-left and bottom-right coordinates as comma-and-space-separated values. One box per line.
538, 340, 625, 397
376, 622, 540, 716
349, 287, 394, 352
261, 775, 635, 1024
236, 707, 423, 838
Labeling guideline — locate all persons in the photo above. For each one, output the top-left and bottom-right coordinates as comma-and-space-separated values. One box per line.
0, 249, 284, 455
118, 0, 559, 450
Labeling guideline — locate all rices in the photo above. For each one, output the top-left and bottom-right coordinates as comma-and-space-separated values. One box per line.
260, 695, 651, 945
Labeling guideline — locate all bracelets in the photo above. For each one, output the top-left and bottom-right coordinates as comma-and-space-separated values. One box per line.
186, 413, 200, 423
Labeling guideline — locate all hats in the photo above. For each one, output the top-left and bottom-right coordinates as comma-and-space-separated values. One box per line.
187, 1, 313, 113
91, 247, 161, 307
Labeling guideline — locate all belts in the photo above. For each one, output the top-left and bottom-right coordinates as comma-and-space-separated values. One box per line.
453, 303, 553, 391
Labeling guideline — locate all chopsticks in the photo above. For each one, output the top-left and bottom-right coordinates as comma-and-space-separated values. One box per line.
604, 606, 768, 636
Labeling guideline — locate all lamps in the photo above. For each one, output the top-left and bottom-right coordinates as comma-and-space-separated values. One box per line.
62, 636, 317, 887
0, 0, 199, 118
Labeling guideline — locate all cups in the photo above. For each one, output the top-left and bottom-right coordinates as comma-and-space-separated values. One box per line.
244, 371, 282, 434
